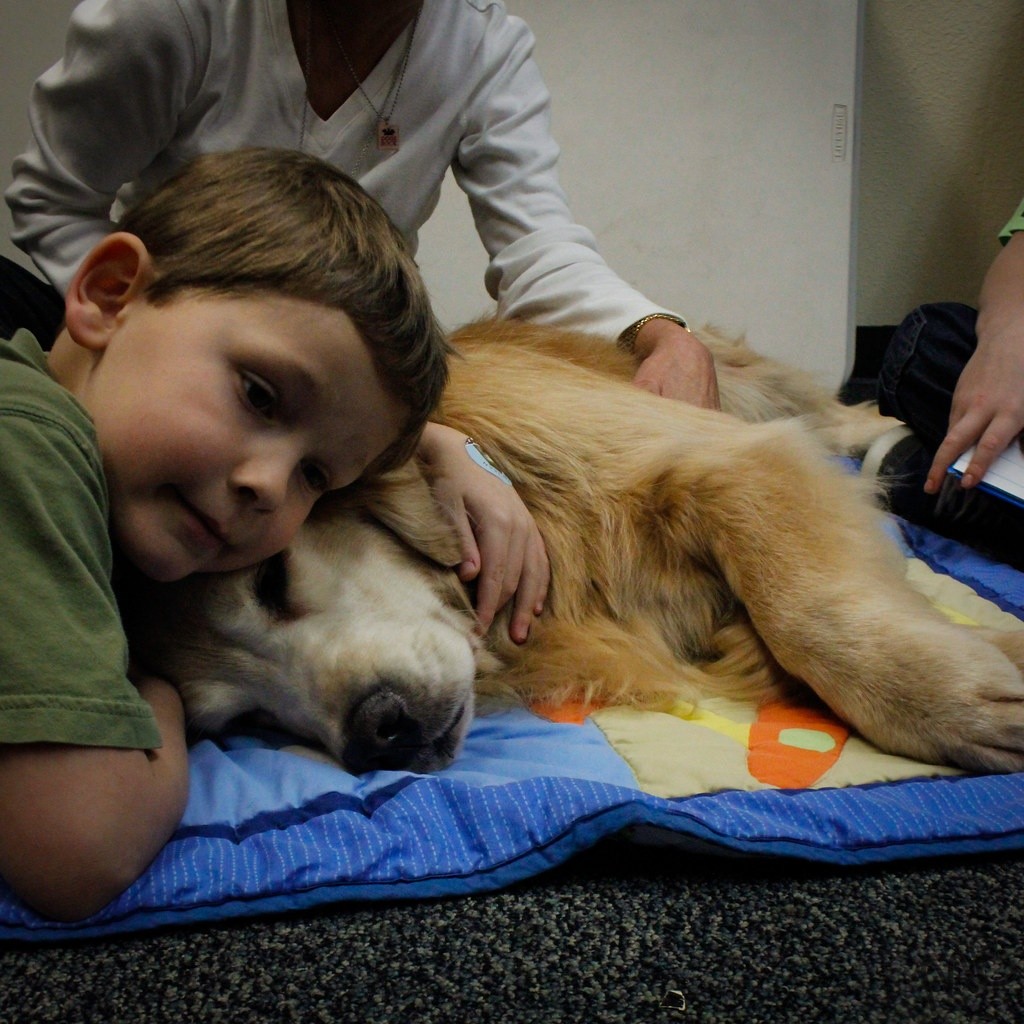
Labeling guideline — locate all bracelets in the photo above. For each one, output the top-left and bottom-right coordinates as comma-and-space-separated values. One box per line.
618, 313, 690, 359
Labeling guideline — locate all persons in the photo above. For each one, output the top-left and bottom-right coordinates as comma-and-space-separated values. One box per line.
1, 0, 721, 412
860, 192, 1023, 574
0, 141, 552, 923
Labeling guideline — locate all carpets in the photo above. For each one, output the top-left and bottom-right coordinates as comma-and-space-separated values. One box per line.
0, 457, 1024, 945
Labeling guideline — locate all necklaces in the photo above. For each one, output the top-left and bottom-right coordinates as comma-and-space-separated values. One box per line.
300, 0, 421, 182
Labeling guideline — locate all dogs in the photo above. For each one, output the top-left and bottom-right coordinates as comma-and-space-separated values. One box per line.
154, 317, 1023, 777
